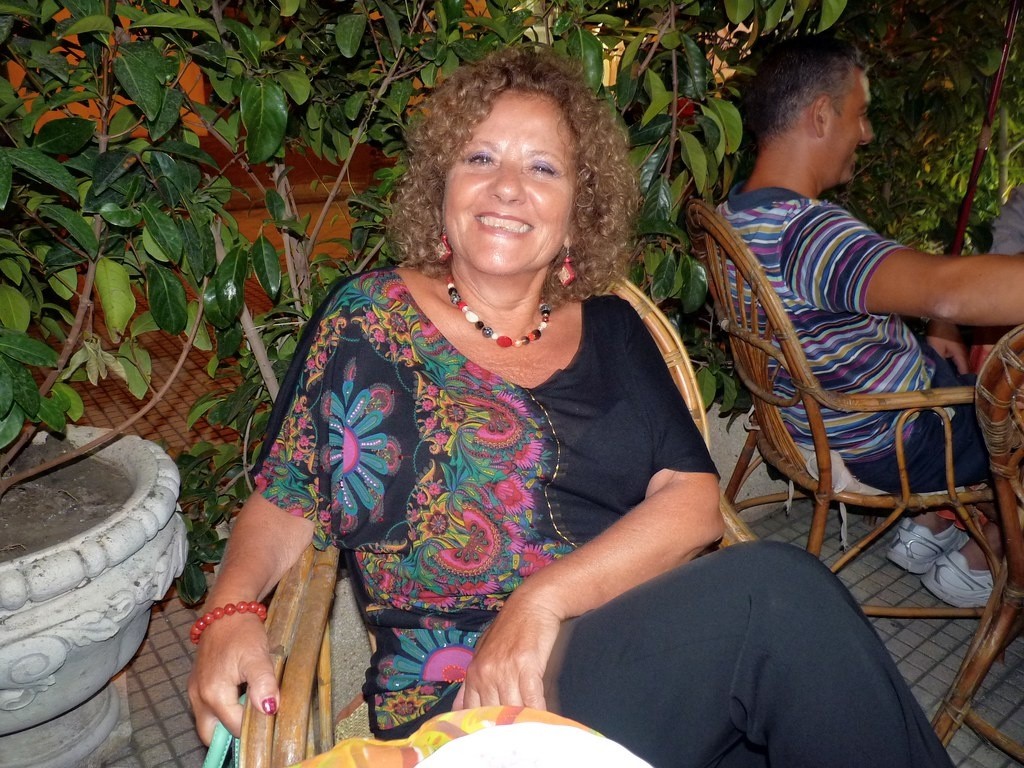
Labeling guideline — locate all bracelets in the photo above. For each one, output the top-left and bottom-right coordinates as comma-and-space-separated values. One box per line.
190, 602, 267, 645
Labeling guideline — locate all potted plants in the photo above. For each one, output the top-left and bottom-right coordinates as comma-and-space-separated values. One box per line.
0, 1, 1023, 767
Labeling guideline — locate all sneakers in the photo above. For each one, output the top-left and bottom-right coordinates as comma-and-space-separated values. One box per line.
921, 549, 993, 608
887, 516, 970, 574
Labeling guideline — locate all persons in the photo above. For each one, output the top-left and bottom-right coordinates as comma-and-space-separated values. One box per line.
188, 44, 959, 768
709, 40, 1024, 607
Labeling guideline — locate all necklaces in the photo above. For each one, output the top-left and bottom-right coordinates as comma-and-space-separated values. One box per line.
443, 275, 552, 350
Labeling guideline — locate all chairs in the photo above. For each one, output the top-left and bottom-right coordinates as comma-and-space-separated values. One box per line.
239, 275, 760, 767
931, 323, 1023, 768
684, 201, 1003, 618
